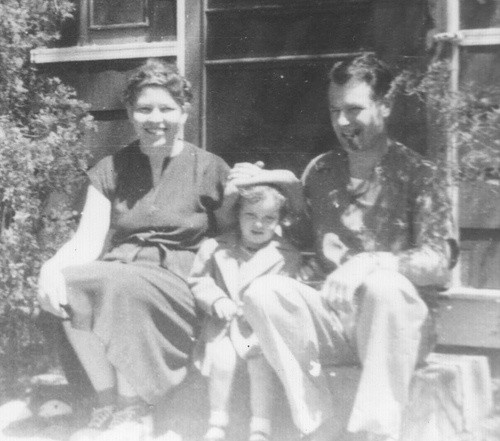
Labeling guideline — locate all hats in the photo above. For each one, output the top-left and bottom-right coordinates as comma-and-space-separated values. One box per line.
213, 155, 306, 233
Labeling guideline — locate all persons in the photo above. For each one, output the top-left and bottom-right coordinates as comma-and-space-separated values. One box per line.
38, 58, 264, 441
189, 169, 305, 441
243, 56, 460, 441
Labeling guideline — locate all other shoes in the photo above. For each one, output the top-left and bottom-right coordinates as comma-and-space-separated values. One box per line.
304, 421, 385, 440
68, 399, 154, 441
204, 423, 270, 440
